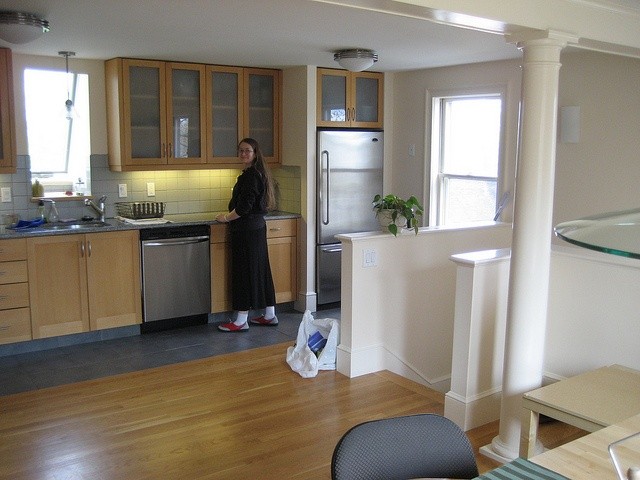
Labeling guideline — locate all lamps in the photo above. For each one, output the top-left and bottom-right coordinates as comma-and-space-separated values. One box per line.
333, 49, 378, 73
61, 50, 81, 119
0, 10, 50, 45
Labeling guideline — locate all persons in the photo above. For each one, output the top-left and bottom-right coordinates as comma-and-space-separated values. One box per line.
218, 138, 279, 332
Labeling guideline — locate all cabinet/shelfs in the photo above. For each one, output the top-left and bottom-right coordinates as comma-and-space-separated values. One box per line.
29, 232, 140, 353
316, 69, 385, 127
209, 220, 298, 323
0, 238, 29, 357
105, 57, 206, 172
0, 46, 17, 174
207, 65, 284, 168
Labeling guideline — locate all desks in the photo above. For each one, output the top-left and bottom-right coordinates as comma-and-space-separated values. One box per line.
471, 412, 640, 480
519, 364, 640, 461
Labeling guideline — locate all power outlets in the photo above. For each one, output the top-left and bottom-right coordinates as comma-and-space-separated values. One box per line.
408, 143, 416, 156
119, 184, 127, 197
361, 246, 378, 269
146, 183, 155, 196
1, 187, 12, 203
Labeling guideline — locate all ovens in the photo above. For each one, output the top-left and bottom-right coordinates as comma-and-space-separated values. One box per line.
141, 227, 210, 334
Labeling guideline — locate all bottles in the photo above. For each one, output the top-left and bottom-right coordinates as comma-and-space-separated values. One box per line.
45, 201, 61, 223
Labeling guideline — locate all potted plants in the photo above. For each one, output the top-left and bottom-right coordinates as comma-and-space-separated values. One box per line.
369, 194, 424, 237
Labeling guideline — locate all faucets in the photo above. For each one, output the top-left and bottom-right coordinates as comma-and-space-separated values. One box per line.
83, 195, 107, 222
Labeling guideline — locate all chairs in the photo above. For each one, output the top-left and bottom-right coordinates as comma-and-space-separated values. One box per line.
331, 413, 479, 480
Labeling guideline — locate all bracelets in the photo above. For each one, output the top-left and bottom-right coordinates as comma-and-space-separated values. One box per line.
223, 212, 230, 223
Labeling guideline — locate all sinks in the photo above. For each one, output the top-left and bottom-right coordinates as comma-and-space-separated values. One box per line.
39, 221, 112, 230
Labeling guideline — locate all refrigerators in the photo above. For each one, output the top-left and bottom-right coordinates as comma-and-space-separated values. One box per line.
320, 133, 387, 309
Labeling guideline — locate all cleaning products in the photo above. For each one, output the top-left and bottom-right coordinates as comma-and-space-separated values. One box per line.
35, 199, 48, 221
31, 177, 44, 198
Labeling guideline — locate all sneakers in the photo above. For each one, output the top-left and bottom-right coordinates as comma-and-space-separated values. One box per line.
218, 321, 249, 331
249, 315, 279, 324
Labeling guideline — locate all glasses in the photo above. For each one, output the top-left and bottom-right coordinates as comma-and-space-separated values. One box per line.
238, 149, 255, 153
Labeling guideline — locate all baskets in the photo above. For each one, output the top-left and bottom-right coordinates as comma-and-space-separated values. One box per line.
114, 200, 166, 219
